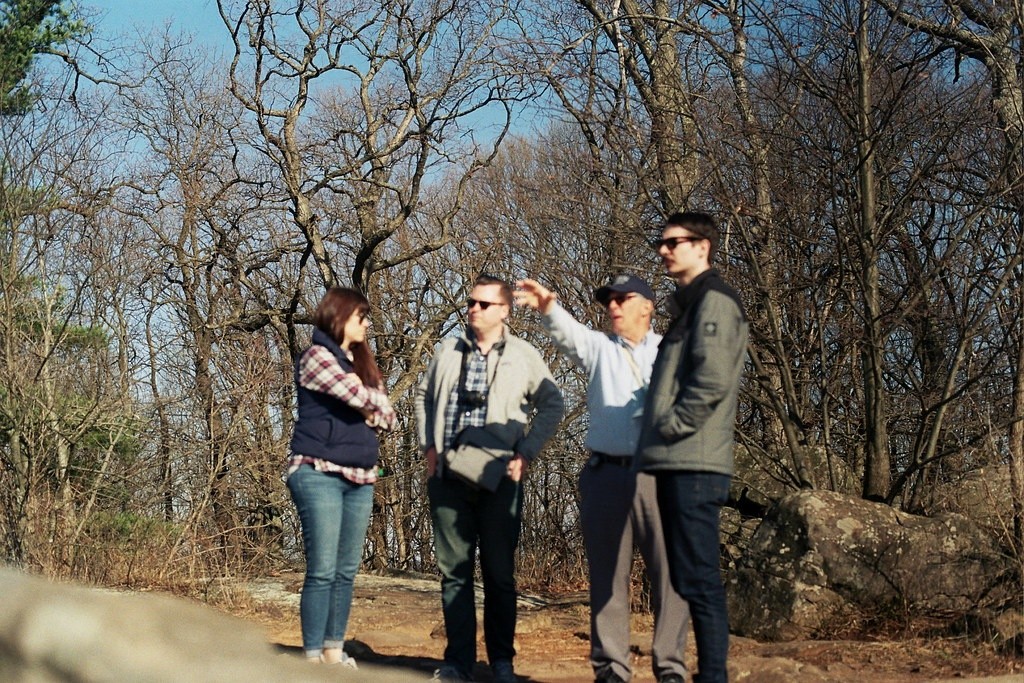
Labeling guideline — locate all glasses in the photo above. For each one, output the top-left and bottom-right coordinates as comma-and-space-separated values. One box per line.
467, 299, 505, 310
652, 236, 702, 250
605, 295, 636, 309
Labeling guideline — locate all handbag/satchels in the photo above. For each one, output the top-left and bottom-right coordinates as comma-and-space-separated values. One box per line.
443, 425, 514, 494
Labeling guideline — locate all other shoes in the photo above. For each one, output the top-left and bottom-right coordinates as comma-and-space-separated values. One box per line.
594, 668, 624, 683
319, 654, 358, 671
491, 659, 517, 683
433, 666, 474, 683
662, 673, 683, 683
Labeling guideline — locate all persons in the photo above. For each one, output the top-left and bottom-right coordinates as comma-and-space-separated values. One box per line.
284, 286, 394, 671
635, 211, 749, 683
513, 273, 690, 683
413, 275, 565, 682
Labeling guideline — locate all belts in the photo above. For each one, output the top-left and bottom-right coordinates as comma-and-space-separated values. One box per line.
593, 452, 633, 466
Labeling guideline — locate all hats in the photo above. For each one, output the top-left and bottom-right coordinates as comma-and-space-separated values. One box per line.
595, 275, 653, 307
